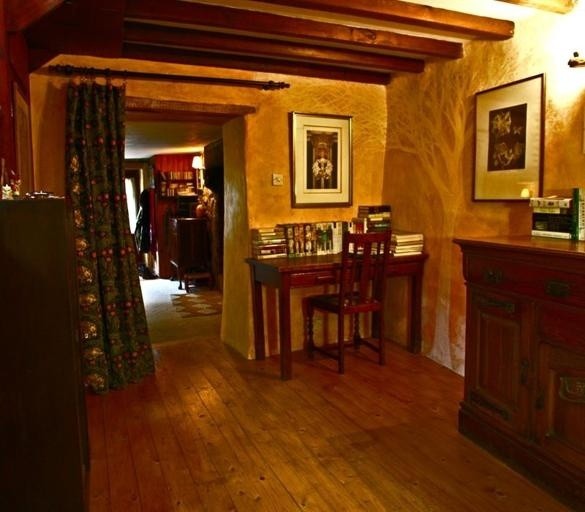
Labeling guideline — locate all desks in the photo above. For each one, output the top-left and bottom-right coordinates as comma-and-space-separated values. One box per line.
169, 290, 225, 319
165, 217, 214, 294
244, 253, 431, 381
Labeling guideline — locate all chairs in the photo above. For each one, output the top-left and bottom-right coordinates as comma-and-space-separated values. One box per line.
306, 226, 398, 375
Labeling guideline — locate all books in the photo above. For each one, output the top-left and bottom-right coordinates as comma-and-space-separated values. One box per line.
158, 171, 196, 197
530, 186, 585, 242
250, 204, 425, 259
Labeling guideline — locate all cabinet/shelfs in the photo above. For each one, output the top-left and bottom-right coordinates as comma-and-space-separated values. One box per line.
144, 152, 200, 278
0, 195, 96, 511
447, 233, 585, 511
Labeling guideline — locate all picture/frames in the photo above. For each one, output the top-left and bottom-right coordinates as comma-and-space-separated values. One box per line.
287, 110, 355, 211
470, 70, 547, 208
